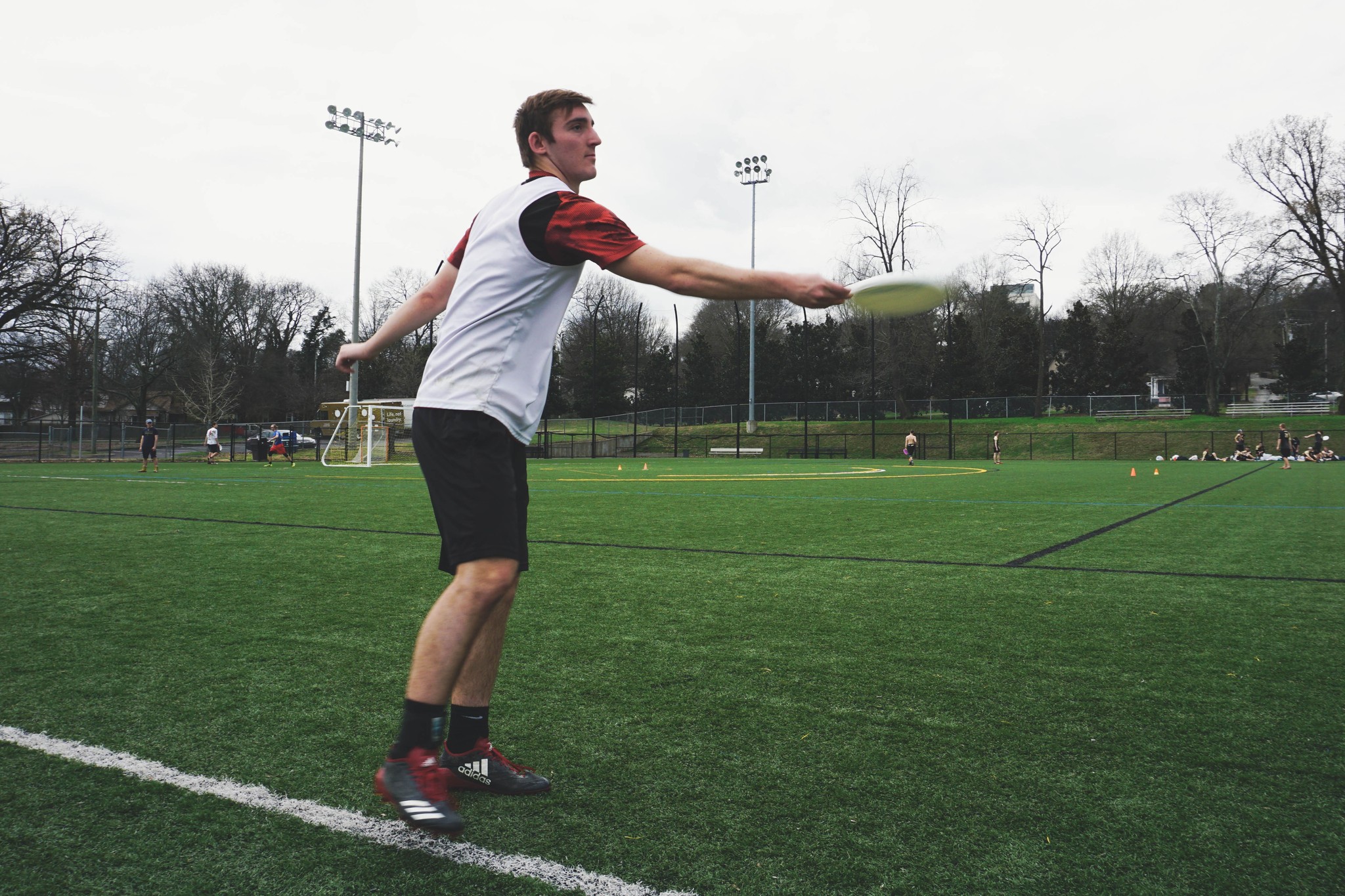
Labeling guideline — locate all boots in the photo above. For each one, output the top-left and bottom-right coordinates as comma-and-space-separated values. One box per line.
138, 465, 147, 472
153, 466, 158, 472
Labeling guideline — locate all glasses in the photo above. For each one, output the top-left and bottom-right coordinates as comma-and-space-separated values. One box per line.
271, 427, 274, 428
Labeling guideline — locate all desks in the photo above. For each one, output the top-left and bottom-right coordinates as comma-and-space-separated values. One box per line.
267, 440, 294, 454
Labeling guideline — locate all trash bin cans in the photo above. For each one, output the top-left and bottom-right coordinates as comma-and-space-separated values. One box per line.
250, 438, 268, 460
282, 431, 297, 453
682, 449, 690, 458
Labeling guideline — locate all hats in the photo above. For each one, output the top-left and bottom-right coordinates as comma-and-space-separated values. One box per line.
146, 419, 152, 423
217, 445, 223, 453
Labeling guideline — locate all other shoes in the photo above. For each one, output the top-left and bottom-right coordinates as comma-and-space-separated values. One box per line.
208, 457, 211, 464
211, 462, 218, 464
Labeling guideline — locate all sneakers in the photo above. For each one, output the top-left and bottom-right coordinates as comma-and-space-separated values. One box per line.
439, 739, 551, 795
292, 462, 295, 467
376, 745, 466, 837
264, 464, 272, 466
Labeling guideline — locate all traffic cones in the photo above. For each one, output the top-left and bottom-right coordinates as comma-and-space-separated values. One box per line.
1154, 468, 1159, 475
618, 465, 622, 470
1174, 458, 1177, 461
1130, 468, 1136, 476
644, 463, 648, 470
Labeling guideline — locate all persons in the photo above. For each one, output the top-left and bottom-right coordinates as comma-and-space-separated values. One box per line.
204, 422, 221, 465
993, 431, 1003, 464
263, 424, 295, 467
137, 419, 158, 472
331, 88, 850, 834
1199, 423, 1345, 470
905, 430, 918, 466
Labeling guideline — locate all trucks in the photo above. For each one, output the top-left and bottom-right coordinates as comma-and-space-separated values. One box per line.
310, 397, 416, 438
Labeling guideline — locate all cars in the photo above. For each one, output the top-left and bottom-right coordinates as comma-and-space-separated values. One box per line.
246, 430, 317, 452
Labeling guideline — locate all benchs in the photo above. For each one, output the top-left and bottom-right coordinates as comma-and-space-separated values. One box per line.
1225, 402, 1331, 419
787, 449, 847, 459
270, 445, 299, 453
1093, 409, 1192, 422
526, 447, 544, 458
708, 448, 763, 458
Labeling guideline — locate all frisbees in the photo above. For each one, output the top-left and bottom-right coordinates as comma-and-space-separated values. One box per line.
842, 269, 944, 320
1322, 435, 1329, 441
903, 449, 908, 455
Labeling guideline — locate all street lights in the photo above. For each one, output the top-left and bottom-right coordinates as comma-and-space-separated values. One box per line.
733, 154, 773, 433
323, 104, 402, 448
1325, 309, 1336, 400
91, 296, 110, 453
